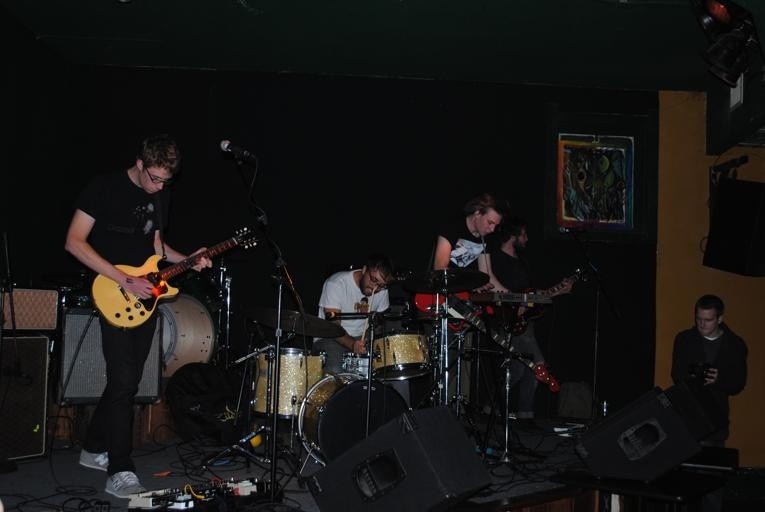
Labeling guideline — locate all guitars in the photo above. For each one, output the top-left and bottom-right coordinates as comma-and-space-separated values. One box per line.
90, 225, 258, 328
500, 263, 601, 336
414, 292, 559, 392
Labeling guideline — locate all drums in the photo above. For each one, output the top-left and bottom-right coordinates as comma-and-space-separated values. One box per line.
298, 372, 409, 465
366, 329, 431, 376
251, 348, 327, 420
340, 352, 370, 373
154, 294, 216, 384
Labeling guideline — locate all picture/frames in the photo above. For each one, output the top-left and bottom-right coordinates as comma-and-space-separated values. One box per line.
555, 133, 634, 232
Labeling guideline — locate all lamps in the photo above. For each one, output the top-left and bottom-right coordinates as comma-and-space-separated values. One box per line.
691, 0, 760, 88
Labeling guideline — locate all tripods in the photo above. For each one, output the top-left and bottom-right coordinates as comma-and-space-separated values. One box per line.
409, 293, 531, 482
190, 260, 305, 504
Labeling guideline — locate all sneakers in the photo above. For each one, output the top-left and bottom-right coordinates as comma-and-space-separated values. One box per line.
105, 471, 146, 498
79, 449, 108, 472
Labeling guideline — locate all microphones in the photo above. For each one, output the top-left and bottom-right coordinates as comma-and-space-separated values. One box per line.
220, 138, 250, 158
558, 226, 588, 235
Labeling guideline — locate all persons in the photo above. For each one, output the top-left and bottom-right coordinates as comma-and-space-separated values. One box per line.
63, 135, 211, 498
428, 190, 523, 421
671, 294, 747, 512
484, 216, 576, 435
311, 255, 410, 408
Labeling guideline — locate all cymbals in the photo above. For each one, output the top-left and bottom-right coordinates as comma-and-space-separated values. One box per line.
257, 307, 346, 339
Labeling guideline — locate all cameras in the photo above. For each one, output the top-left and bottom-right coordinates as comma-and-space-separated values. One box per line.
685, 359, 714, 381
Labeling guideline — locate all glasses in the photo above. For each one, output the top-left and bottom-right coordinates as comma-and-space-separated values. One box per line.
369, 271, 387, 290
146, 168, 168, 184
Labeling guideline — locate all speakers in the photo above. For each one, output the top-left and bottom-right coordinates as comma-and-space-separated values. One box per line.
307, 404, 495, 512
582, 379, 727, 484
